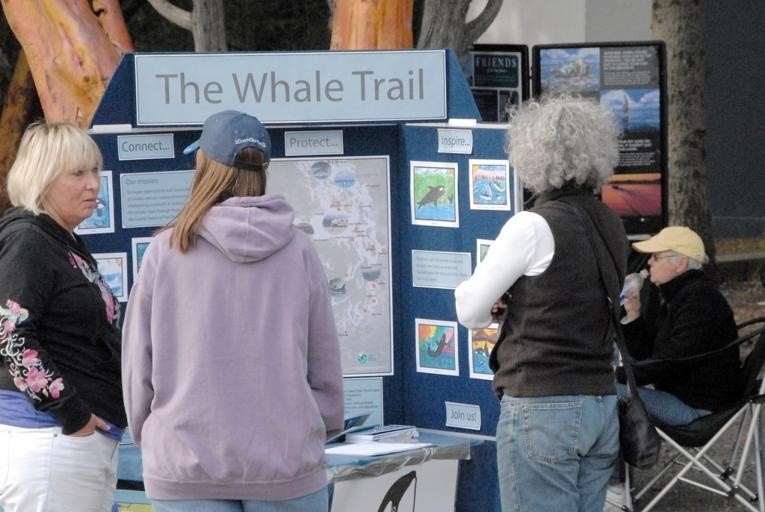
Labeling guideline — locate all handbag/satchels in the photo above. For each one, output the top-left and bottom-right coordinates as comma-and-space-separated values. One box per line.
618, 384, 662, 472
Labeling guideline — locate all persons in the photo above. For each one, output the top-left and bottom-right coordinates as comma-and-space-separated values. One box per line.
606, 226, 740, 511
450, 93, 630, 512
120, 111, 344, 512
0, 123, 129, 512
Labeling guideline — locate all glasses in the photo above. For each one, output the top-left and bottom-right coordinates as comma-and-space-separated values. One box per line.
652, 254, 677, 261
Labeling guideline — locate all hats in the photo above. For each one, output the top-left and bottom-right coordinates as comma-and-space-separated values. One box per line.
182, 109, 272, 172
632, 226, 708, 264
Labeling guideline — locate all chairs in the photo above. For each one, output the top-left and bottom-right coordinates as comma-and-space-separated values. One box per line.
374, 469, 419, 512
615, 308, 760, 512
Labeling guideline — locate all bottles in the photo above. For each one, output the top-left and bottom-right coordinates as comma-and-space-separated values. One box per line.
619, 269, 650, 306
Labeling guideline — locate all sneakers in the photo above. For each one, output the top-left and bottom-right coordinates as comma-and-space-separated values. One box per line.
607, 487, 635, 510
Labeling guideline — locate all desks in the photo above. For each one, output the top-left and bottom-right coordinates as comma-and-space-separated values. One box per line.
316, 428, 471, 512
